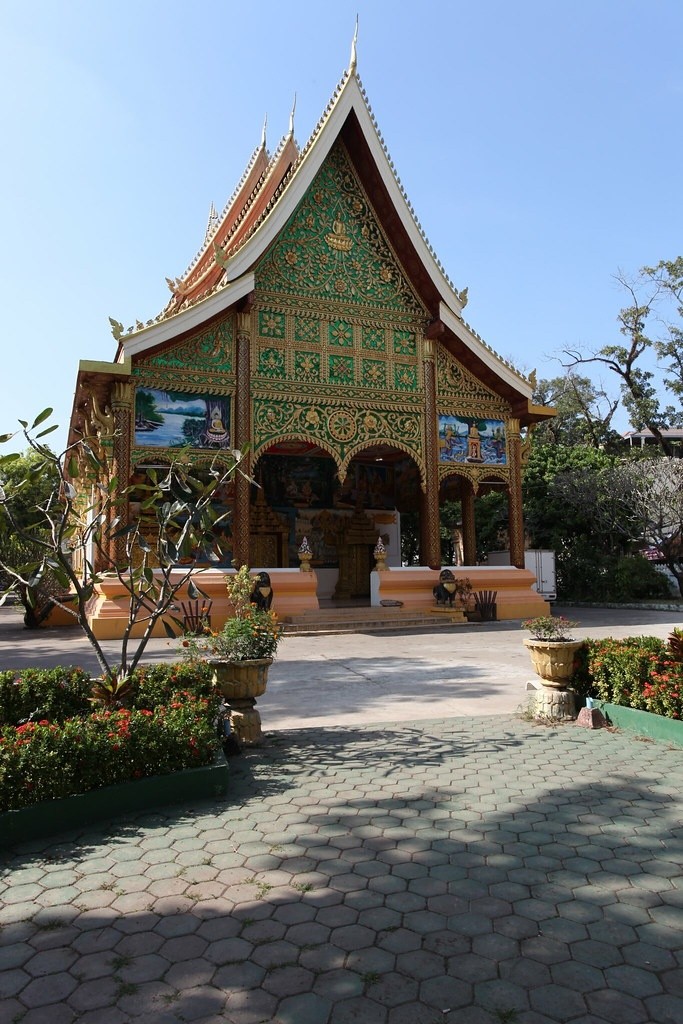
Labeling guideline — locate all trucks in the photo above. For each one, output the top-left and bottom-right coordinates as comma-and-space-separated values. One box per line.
480, 549, 558, 604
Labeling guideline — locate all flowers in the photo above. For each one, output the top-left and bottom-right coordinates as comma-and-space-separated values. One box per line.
184, 566, 284, 659
456, 577, 474, 613
521, 615, 581, 641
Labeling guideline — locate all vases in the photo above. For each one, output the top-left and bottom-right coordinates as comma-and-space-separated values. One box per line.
464, 610, 482, 621
523, 639, 583, 686
206, 657, 273, 698
